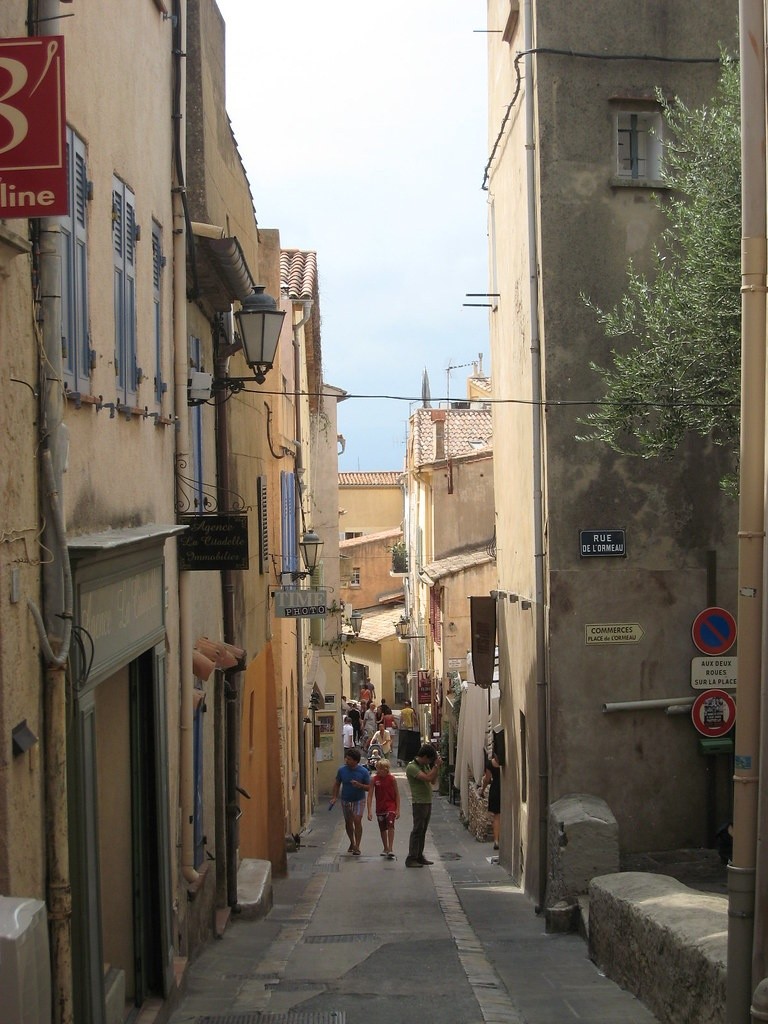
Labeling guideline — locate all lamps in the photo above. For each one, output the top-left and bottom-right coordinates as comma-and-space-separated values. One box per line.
279, 526, 326, 586
212, 285, 289, 389
395, 616, 425, 639
343, 609, 363, 639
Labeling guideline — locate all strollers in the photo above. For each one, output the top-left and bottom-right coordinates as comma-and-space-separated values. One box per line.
367, 744, 386, 771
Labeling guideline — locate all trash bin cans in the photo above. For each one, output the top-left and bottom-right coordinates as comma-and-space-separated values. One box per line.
396, 728, 421, 767
695, 737, 734, 864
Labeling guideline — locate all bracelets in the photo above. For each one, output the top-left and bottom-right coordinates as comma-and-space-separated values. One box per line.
433, 763, 439, 768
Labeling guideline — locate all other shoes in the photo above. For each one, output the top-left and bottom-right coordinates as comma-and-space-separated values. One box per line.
388, 852, 395, 857
406, 861, 423, 867
348, 843, 355, 852
353, 849, 361, 855
380, 850, 388, 856
494, 844, 499, 850
418, 857, 434, 865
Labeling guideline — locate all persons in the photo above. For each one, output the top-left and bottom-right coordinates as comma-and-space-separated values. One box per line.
367, 757, 400, 858
479, 757, 501, 850
342, 677, 399, 767
399, 701, 415, 731
329, 749, 371, 855
405, 744, 443, 868
